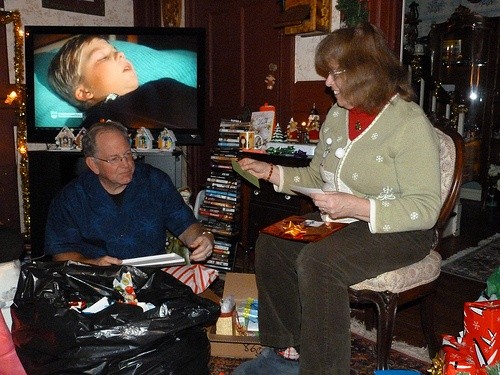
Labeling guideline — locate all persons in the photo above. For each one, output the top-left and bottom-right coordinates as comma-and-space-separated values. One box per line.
42, 120, 217, 268
232, 21, 442, 375
47, 34, 198, 129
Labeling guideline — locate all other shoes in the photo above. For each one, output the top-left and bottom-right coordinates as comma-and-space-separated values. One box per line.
231, 347, 300, 375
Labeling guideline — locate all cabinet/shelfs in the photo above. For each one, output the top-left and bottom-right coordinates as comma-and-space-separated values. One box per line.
235, 150, 315, 250
427, 5, 500, 211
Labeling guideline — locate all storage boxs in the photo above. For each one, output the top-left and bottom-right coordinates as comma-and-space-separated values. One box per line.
198, 272, 261, 358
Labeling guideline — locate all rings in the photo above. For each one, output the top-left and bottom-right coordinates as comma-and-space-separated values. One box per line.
207, 251, 211, 257
321, 207, 325, 214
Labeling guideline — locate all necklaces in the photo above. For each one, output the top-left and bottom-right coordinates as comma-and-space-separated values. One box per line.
201, 231, 212, 235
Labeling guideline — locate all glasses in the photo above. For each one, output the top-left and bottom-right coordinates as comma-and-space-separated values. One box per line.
93, 147, 137, 166
328, 69, 347, 82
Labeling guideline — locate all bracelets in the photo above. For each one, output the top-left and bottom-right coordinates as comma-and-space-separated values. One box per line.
265, 163, 273, 181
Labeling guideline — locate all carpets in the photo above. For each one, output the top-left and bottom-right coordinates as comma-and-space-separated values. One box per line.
440, 232, 500, 284
207, 310, 433, 375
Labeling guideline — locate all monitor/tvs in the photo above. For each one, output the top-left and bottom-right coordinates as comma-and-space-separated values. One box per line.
25, 25, 207, 145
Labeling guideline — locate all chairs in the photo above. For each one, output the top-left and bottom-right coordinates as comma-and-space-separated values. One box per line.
347, 108, 466, 371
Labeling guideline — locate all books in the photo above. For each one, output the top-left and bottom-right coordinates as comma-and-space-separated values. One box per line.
197, 119, 256, 271
120, 253, 186, 266
